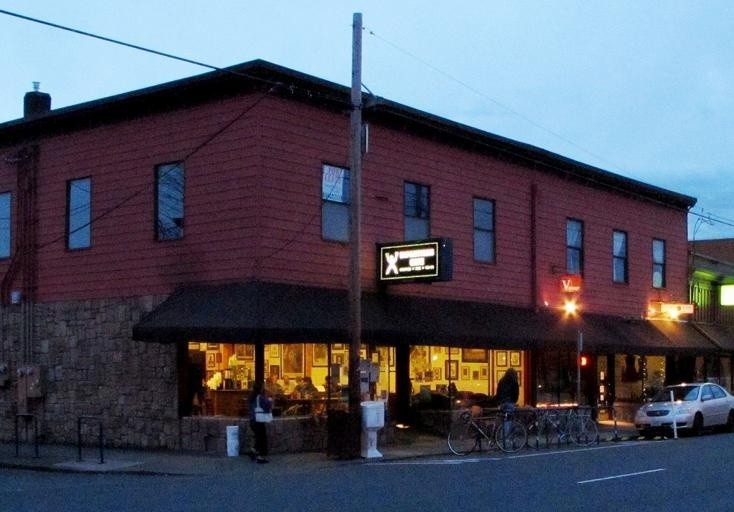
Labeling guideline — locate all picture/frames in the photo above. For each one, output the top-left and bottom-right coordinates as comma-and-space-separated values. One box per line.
204, 341, 522, 386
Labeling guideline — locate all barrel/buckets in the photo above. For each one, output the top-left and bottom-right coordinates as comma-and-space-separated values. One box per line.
225, 425, 240, 440
226, 441, 240, 457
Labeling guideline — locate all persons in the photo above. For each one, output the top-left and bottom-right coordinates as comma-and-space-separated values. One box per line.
494, 368, 520, 406
267, 371, 339, 419
244, 379, 273, 464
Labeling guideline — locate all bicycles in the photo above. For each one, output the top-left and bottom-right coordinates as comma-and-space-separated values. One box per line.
447, 406, 599, 456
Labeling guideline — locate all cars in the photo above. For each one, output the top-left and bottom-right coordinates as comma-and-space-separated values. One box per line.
634, 383, 734, 437
